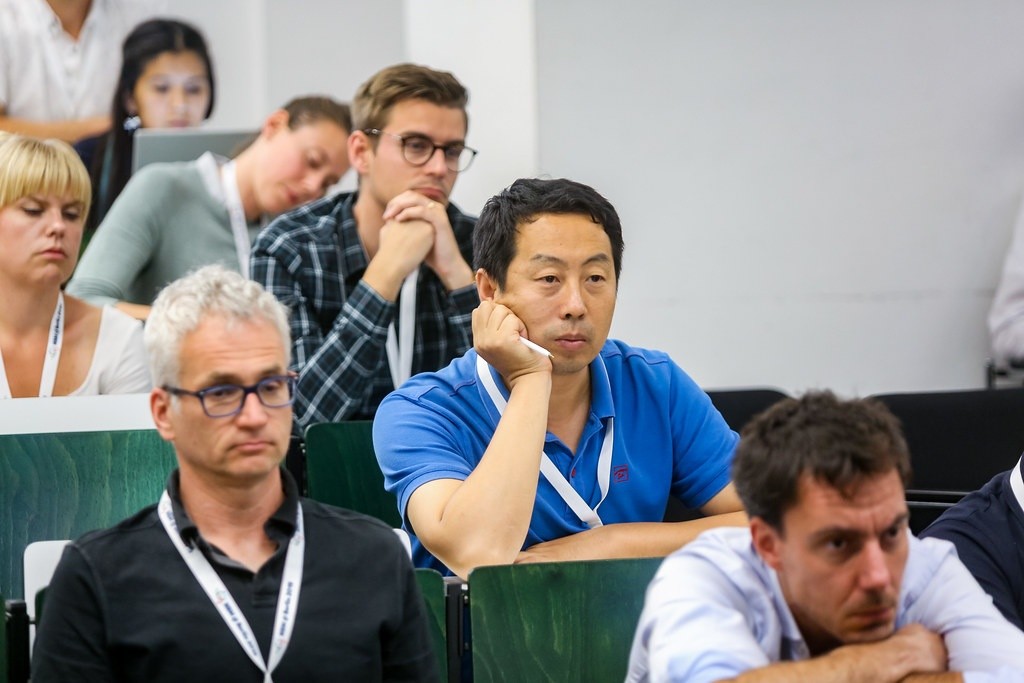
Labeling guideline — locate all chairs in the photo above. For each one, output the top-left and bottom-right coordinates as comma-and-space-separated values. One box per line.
703, 389, 787, 432
861, 387, 1024, 493
0, 429, 177, 683
446, 556, 662, 683
287, 420, 403, 530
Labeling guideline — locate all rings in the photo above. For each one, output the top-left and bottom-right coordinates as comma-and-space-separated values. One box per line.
428, 202, 434, 209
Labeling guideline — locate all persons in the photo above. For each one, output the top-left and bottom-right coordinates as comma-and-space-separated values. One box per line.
63, 95, 354, 325
248, 60, 485, 440
0, 0, 126, 143
988, 203, 1024, 389
923, 454, 1024, 632
1, 133, 154, 400
624, 388, 1024, 683
372, 178, 750, 585
32, 263, 453, 683
59, 17, 217, 304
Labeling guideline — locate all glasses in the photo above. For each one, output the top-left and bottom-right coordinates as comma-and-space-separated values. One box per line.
163, 371, 299, 417
365, 128, 477, 173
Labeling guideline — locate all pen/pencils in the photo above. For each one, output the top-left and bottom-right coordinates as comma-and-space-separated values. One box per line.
520, 336, 554, 358
